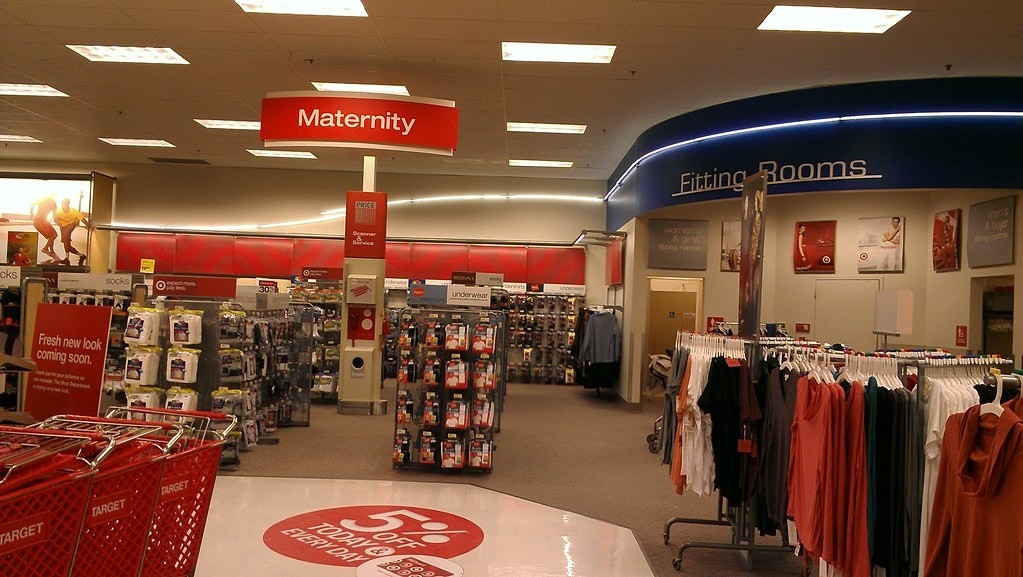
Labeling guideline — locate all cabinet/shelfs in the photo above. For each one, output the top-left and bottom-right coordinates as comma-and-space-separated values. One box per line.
0, 264, 344, 471
392, 308, 514, 473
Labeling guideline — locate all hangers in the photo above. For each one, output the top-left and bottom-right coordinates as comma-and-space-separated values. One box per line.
675, 330, 1023, 419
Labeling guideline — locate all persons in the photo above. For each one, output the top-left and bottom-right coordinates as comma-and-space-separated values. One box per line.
729, 242, 741, 269
876, 217, 900, 270
30, 198, 61, 262
13, 245, 31, 265
937, 216, 956, 269
442, 335, 486, 468
795, 224, 812, 271
55, 199, 93, 266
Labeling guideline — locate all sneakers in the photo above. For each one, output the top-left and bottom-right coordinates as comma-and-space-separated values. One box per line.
61, 258, 70, 266
78, 254, 86, 266
49, 252, 62, 262
41, 248, 50, 256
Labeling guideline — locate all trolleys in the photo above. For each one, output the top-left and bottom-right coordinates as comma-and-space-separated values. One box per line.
0, 406, 237, 577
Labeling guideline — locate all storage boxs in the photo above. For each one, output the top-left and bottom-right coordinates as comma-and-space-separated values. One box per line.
441, 323, 498, 470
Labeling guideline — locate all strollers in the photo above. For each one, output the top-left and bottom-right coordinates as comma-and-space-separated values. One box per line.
647, 354, 672, 453
